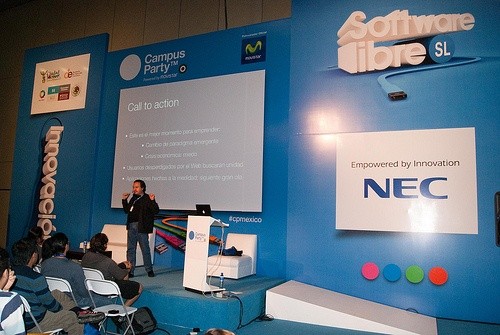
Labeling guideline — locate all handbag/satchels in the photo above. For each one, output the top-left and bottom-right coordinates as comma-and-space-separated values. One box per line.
123, 307, 158, 335
219, 246, 242, 256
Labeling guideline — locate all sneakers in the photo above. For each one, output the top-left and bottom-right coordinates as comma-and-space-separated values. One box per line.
77, 306, 105, 324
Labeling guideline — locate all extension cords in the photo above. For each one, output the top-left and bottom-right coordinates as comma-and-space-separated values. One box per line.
190, 331, 201, 335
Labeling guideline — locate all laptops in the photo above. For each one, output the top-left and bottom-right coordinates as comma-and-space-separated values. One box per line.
195, 204, 212, 217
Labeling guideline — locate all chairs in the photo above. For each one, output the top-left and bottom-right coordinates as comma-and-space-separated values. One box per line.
208, 233, 258, 279
19, 267, 138, 335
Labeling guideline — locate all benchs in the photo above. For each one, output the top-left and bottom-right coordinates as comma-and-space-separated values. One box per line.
100, 223, 156, 266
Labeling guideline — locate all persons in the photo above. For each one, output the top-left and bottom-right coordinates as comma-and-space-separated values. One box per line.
0, 226, 94, 335
80, 233, 143, 307
204, 328, 236, 335
122, 180, 160, 278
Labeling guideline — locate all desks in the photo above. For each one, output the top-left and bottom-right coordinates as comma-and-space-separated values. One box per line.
65, 246, 112, 262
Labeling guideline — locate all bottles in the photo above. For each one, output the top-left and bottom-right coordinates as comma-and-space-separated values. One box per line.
219, 273, 224, 288
83, 238, 87, 250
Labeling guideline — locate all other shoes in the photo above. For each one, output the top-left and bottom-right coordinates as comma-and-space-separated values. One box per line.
148, 270, 154, 277
129, 274, 134, 278
